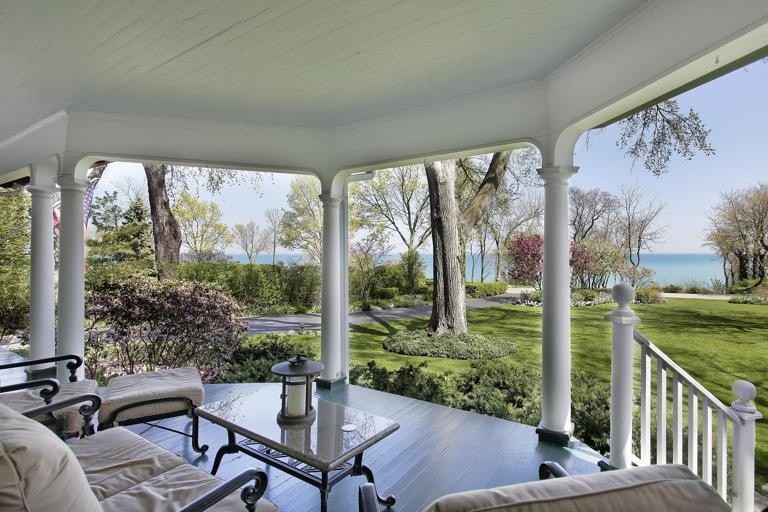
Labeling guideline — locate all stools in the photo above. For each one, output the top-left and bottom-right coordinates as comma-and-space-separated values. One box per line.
97, 366, 209, 452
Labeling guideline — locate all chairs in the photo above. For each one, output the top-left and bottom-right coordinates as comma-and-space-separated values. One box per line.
0, 354, 102, 455
358, 461, 731, 512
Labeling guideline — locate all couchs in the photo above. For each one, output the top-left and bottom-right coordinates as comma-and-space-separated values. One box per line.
0, 402, 279, 512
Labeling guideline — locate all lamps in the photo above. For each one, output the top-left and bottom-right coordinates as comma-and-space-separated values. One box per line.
271, 353, 325, 424
278, 424, 312, 456
